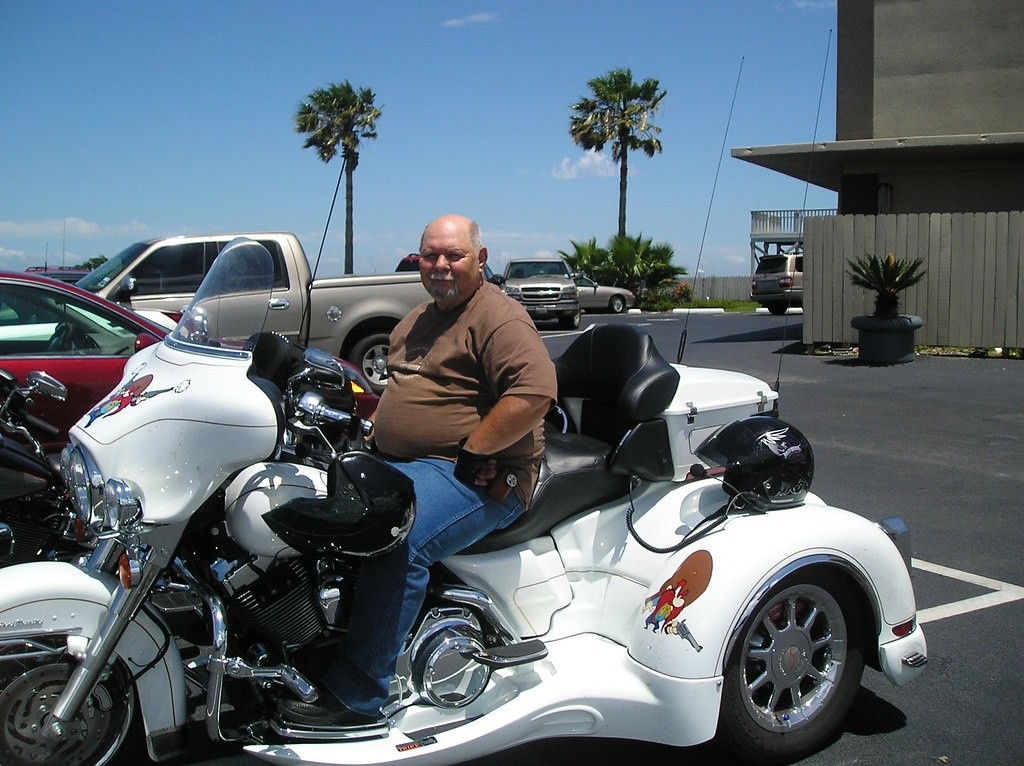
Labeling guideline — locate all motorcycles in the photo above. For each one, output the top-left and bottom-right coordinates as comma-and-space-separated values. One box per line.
1, 235, 930, 765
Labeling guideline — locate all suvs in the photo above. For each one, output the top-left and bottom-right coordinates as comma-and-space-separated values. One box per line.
749, 252, 805, 316
394, 253, 504, 286
501, 257, 585, 328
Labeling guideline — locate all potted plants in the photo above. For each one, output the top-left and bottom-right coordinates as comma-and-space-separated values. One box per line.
843, 250, 931, 365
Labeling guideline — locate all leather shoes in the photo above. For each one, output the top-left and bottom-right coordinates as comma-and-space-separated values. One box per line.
277, 683, 377, 727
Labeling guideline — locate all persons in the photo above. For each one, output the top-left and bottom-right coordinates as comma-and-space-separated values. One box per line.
277, 214, 557, 725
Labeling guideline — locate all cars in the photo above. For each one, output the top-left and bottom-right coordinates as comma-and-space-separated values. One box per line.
571, 274, 636, 313
1, 268, 376, 452
0, 285, 194, 349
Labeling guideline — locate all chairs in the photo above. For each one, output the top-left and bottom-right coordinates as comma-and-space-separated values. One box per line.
526, 316, 685, 533
549, 268, 558, 273
514, 269, 524, 278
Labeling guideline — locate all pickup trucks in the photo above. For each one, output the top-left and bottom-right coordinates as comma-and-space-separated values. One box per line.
76, 232, 433, 397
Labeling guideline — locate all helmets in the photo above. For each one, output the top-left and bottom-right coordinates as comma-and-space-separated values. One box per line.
262, 450, 418, 560
694, 416, 814, 508
